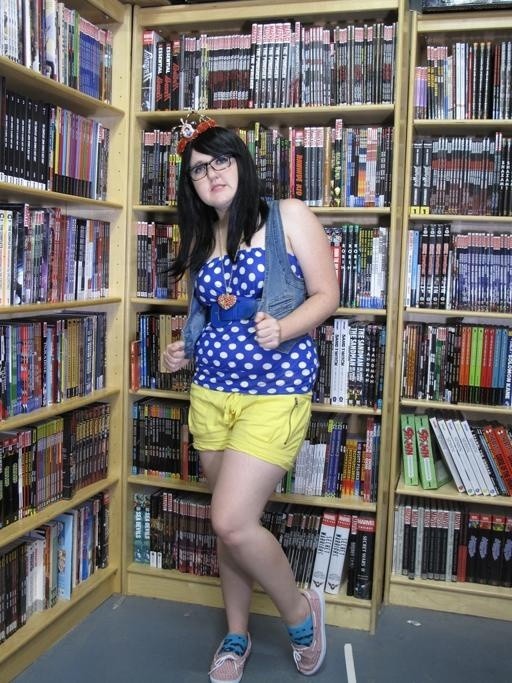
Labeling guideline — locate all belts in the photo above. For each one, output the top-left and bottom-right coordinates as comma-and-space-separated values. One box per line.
205, 295, 263, 328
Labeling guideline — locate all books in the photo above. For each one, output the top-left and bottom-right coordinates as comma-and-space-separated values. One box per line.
310, 318, 386, 410
0, 0, 115, 105
411, 132, 512, 216
260, 500, 376, 600
405, 223, 512, 315
400, 319, 512, 406
322, 224, 389, 308
0, 310, 107, 419
275, 411, 381, 504
0, 490, 109, 645
137, 219, 189, 302
0, 202, 111, 306
238, 118, 393, 208
393, 495, 512, 587
0, 76, 110, 200
133, 486, 219, 577
130, 312, 196, 393
141, 20, 396, 112
140, 129, 182, 208
400, 406, 512, 498
414, 41, 512, 120
132, 395, 208, 485
0, 402, 110, 529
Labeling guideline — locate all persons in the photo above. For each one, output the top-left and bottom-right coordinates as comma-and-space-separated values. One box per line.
158, 126, 339, 682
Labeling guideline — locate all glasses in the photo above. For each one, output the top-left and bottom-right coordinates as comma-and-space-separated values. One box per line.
188, 153, 235, 180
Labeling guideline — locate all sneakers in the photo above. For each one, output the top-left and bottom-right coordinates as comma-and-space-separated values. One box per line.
207, 628, 254, 683
289, 586, 329, 677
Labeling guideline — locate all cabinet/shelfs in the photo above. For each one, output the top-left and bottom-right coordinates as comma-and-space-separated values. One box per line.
123, 0, 403, 636
1, 0, 134, 680
384, 1, 511, 621
